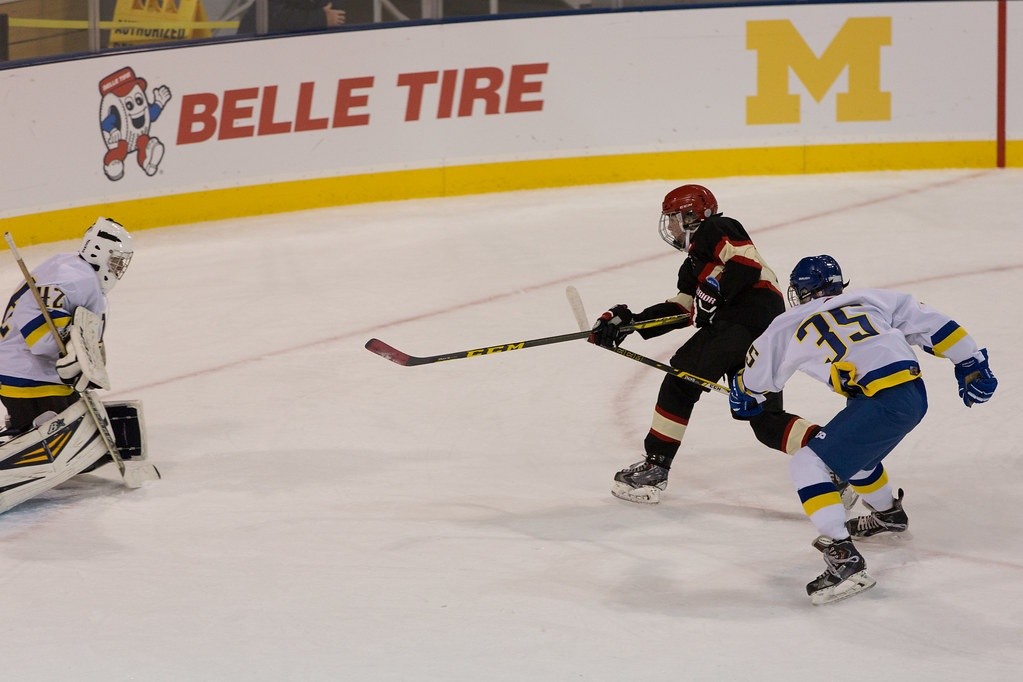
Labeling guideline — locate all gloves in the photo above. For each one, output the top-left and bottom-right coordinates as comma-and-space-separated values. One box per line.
953, 348, 997, 408
590, 304, 635, 349
728, 368, 773, 421
689, 277, 720, 328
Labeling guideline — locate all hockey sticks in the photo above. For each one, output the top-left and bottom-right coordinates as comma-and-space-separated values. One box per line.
2, 231, 163, 483
364, 312, 693, 366
566, 285, 731, 394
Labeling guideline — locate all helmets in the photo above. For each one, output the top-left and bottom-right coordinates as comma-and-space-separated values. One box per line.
659, 184, 718, 253
787, 255, 844, 308
78, 217, 134, 297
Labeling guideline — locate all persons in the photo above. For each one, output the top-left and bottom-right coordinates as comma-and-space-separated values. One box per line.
591, 184, 859, 509
729, 255, 998, 605
0, 217, 147, 512
204, 0, 346, 38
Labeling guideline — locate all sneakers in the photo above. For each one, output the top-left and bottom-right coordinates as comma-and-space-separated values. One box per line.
831, 472, 859, 509
846, 487, 908, 540
807, 535, 876, 605
612, 455, 669, 503
